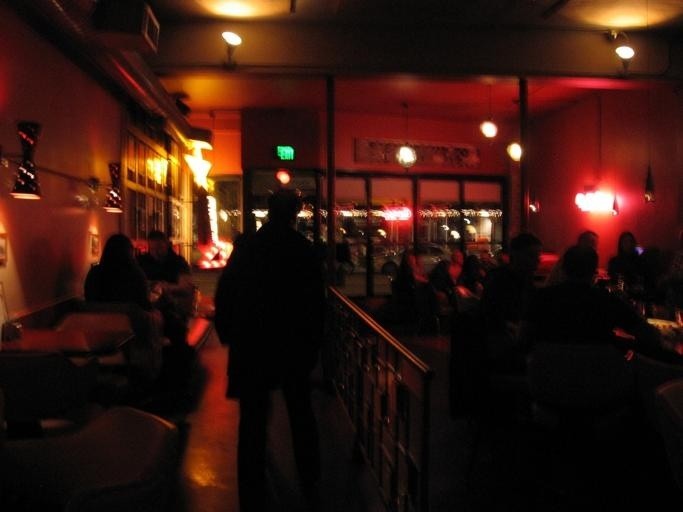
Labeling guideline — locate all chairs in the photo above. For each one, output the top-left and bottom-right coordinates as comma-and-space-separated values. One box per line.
381, 258, 682, 511
2, 270, 212, 511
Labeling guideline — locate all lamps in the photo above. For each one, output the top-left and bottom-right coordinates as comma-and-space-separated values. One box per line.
11, 117, 50, 203
103, 162, 128, 214
639, 93, 658, 204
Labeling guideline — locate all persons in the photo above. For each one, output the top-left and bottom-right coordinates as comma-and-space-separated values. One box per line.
318, 213, 364, 256
85, 223, 197, 346
387, 227, 682, 479
213, 186, 336, 507
